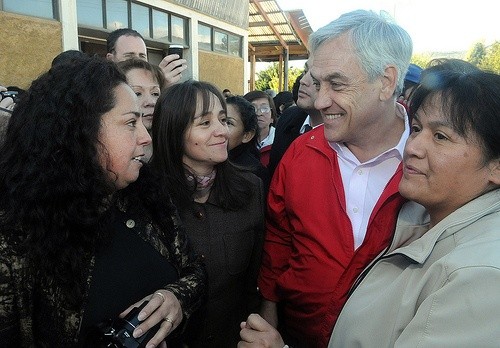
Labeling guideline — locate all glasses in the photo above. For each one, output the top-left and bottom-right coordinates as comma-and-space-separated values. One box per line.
252, 106, 272, 114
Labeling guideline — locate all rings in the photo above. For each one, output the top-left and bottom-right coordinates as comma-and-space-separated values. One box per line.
152, 293, 166, 302
164, 318, 174, 327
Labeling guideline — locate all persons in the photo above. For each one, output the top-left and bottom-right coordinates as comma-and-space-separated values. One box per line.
141, 79, 267, 348
0, 50, 187, 348
236, 70, 500, 348
0, 26, 480, 186
253, 7, 413, 348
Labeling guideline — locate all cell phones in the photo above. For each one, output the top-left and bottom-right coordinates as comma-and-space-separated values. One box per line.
168, 44, 184, 74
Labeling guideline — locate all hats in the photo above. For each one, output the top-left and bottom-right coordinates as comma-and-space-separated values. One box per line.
403, 63, 423, 84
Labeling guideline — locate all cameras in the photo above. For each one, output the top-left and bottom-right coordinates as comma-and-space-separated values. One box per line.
104, 300, 164, 348
0, 86, 25, 104
